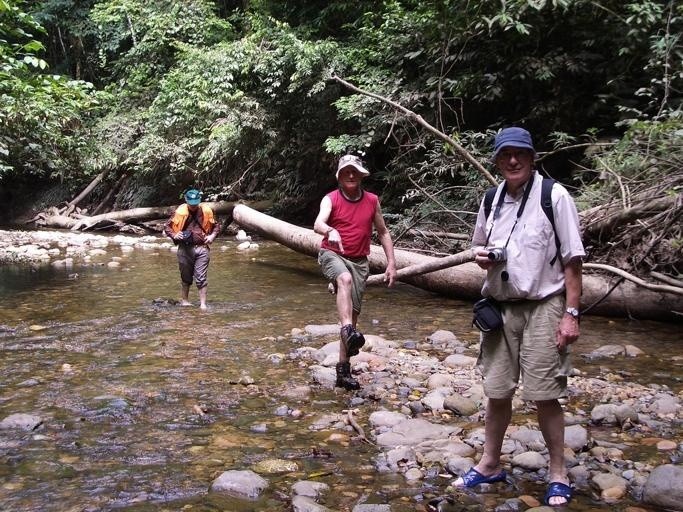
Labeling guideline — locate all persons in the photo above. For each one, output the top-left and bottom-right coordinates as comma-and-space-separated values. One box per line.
164, 189, 220, 311
314, 155, 396, 390
450, 129, 586, 508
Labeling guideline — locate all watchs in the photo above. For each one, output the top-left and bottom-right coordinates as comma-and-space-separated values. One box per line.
564, 308, 579, 318
325, 226, 338, 239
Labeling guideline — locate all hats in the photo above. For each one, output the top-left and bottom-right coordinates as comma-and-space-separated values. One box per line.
187, 190, 200, 205
494, 127, 536, 157
336, 155, 370, 180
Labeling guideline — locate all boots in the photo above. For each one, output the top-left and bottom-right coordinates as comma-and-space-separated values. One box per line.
340, 324, 364, 356
336, 363, 360, 390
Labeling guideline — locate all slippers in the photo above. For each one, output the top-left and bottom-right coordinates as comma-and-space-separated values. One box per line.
454, 468, 506, 487
544, 479, 571, 506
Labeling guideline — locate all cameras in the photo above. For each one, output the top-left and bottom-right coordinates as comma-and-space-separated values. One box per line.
182, 230, 194, 247
486, 247, 506, 262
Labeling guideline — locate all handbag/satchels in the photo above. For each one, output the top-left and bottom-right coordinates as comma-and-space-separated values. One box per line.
472, 298, 504, 333
